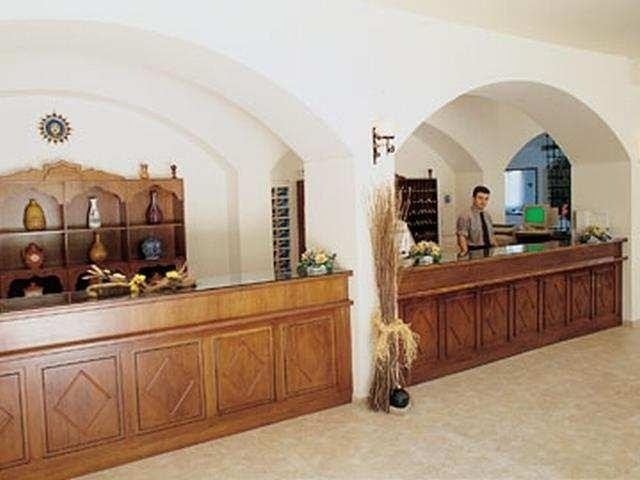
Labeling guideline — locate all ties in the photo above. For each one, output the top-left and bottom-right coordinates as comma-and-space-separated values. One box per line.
479, 212, 491, 247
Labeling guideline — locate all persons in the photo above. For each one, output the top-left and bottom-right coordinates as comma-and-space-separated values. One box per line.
456, 186, 502, 248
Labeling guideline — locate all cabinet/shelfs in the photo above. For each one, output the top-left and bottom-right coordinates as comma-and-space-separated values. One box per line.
297, 177, 439, 257
0, 158, 188, 301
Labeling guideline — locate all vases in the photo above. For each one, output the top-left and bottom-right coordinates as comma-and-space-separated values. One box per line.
22, 192, 164, 269
304, 264, 328, 275
418, 255, 435, 267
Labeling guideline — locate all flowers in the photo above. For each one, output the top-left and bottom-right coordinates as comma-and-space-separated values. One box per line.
410, 239, 441, 263
581, 225, 610, 241
295, 250, 336, 277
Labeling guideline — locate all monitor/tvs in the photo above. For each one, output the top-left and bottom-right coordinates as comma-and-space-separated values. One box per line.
522, 203, 549, 228
